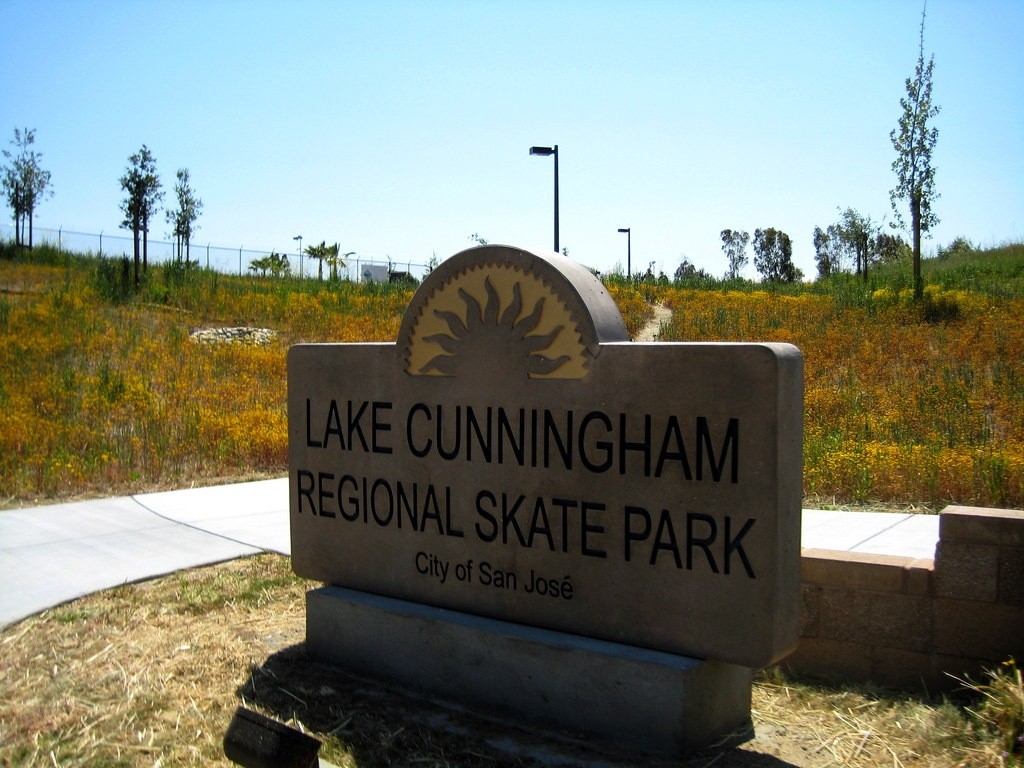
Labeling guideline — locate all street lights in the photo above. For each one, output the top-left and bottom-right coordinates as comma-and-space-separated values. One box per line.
529, 144, 560, 253
617, 228, 631, 281
292, 236, 303, 280
